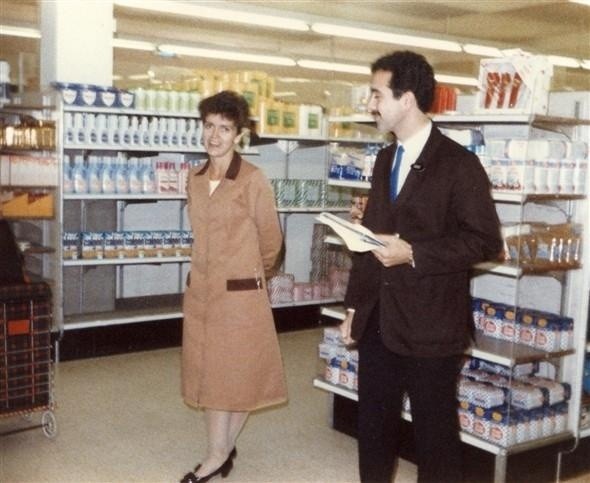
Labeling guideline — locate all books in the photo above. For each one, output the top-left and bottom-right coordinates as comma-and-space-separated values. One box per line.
317, 212, 400, 254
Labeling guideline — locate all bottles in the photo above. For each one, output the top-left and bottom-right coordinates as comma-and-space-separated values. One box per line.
62, 154, 202, 199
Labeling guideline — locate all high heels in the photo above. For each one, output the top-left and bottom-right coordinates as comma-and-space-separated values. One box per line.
179, 446, 236, 483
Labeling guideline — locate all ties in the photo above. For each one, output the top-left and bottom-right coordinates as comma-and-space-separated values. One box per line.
389, 147, 404, 203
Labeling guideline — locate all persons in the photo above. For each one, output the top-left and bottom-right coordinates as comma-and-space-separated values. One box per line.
345, 51, 501, 482
178, 89, 288, 482
